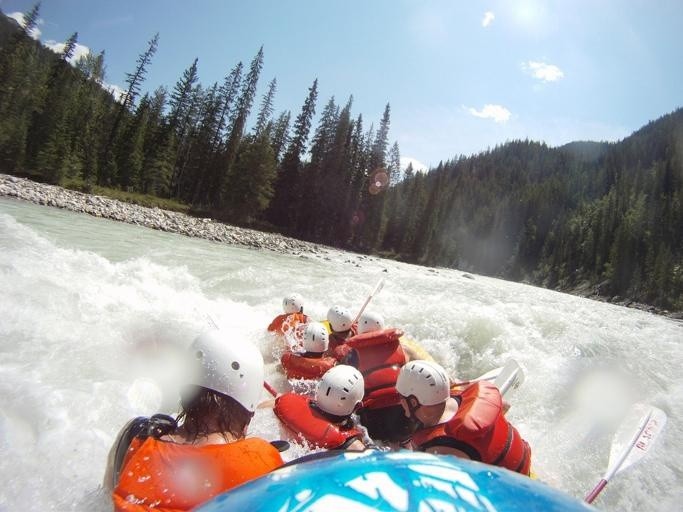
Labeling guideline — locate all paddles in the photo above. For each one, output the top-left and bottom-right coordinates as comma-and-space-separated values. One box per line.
450, 367, 502, 388
494, 366, 525, 405
584, 403, 668, 504
344, 278, 386, 338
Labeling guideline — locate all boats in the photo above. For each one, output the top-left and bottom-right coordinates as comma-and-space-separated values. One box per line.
184, 443, 604, 512
255, 316, 455, 468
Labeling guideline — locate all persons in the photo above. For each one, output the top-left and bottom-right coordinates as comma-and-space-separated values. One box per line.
345, 313, 419, 452
394, 360, 532, 479
277, 323, 339, 383
255, 364, 375, 451
324, 306, 360, 362
266, 293, 313, 333
101, 329, 284, 512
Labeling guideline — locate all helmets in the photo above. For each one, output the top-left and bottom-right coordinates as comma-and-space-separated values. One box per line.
326, 304, 355, 333
394, 359, 451, 407
356, 311, 384, 335
303, 321, 330, 354
178, 327, 266, 417
282, 292, 304, 314
314, 365, 365, 418
